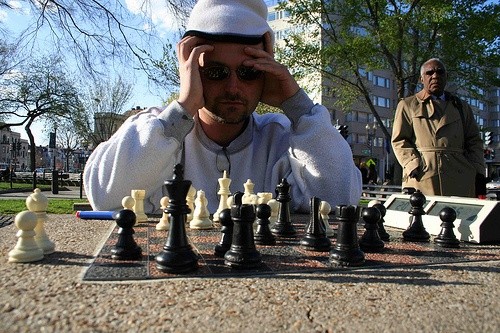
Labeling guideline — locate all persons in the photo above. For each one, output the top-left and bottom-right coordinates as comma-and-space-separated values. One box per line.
360, 163, 377, 198
83, 0, 362, 214
391, 58, 487, 198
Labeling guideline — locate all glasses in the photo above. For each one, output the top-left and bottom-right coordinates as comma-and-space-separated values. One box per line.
215, 145, 232, 202
197, 60, 267, 82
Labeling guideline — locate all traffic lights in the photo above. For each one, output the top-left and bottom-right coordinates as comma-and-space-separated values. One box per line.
17, 143, 22, 150
343, 125, 349, 141
13, 143, 17, 150
336, 125, 343, 136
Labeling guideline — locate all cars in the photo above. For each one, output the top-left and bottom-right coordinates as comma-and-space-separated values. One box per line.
0, 163, 53, 173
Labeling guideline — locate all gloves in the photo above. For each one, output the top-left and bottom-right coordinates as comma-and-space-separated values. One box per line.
408, 167, 426, 183
475, 172, 492, 195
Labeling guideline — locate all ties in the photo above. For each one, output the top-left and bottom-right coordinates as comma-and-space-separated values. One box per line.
435, 98, 445, 121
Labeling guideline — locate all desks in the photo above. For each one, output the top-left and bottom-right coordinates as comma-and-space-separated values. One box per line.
0, 212, 500, 333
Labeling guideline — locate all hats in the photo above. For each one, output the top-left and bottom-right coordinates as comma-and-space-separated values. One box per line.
181, 0, 276, 47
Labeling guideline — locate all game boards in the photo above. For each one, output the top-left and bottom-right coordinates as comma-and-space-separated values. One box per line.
78, 212, 500, 285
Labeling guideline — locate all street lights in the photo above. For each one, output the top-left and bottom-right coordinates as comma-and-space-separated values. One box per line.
53, 121, 57, 171
